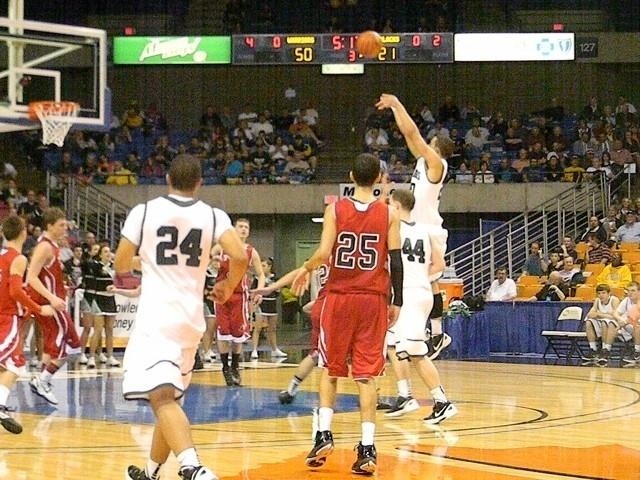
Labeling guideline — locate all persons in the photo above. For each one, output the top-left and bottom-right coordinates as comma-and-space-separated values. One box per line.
287, 154, 406, 475
230, 0, 460, 34
0, 91, 640, 412
386, 192, 456, 429
0, 215, 55, 438
114, 153, 247, 480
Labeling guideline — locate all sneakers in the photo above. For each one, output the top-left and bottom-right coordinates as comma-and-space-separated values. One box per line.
222, 366, 232, 385
305, 430, 335, 471
125, 464, 161, 480
424, 403, 459, 424
278, 388, 297, 404
231, 365, 241, 385
193, 348, 288, 367
29, 374, 58, 404
177, 465, 217, 480
427, 333, 452, 359
30, 351, 121, 368
581, 349, 640, 362
376, 401, 391, 410
385, 394, 422, 419
0, 405, 23, 434
350, 441, 376, 475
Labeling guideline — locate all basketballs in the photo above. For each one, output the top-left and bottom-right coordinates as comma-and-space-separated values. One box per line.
357, 31, 381, 58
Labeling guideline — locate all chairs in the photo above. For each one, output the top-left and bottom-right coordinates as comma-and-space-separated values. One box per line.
513, 241, 640, 366
